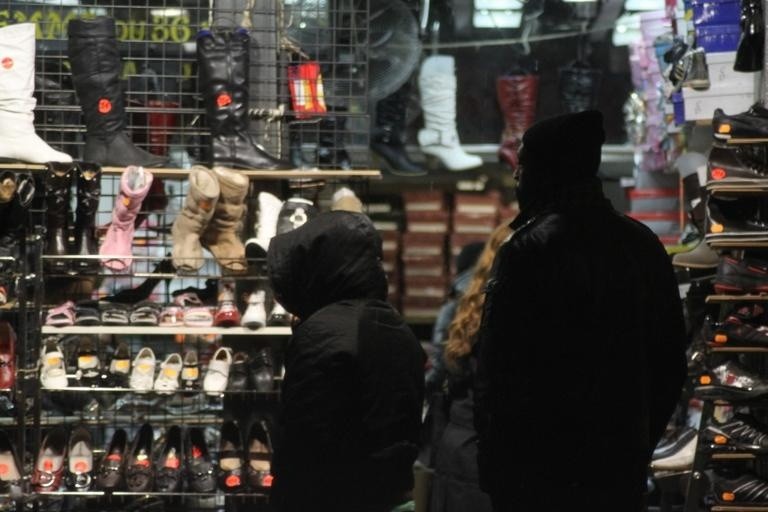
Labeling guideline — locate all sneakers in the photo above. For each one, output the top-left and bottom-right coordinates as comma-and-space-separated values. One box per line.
649, 103, 768, 508
664, 40, 709, 92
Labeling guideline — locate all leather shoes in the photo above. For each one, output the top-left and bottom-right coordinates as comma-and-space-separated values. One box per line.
732, 1, 765, 73
1, 427, 277, 494
1, 280, 299, 422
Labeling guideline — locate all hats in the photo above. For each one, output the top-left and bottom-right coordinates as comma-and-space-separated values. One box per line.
522, 110, 606, 175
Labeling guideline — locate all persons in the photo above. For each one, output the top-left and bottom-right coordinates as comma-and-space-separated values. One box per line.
429, 213, 519, 511
267, 210, 429, 512
425, 240, 486, 393
470, 106, 690, 512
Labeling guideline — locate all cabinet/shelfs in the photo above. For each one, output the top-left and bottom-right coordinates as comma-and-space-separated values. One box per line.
645, 130, 767, 512
0, 165, 382, 512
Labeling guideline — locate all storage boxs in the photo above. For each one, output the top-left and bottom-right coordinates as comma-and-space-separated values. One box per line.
355, 190, 518, 323
669, 0, 760, 126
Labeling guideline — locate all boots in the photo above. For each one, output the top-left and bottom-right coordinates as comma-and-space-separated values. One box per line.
495, 75, 538, 168
202, 29, 295, 170
0, 163, 362, 275
66, 16, 181, 169
317, 57, 483, 178
0, 22, 72, 163
